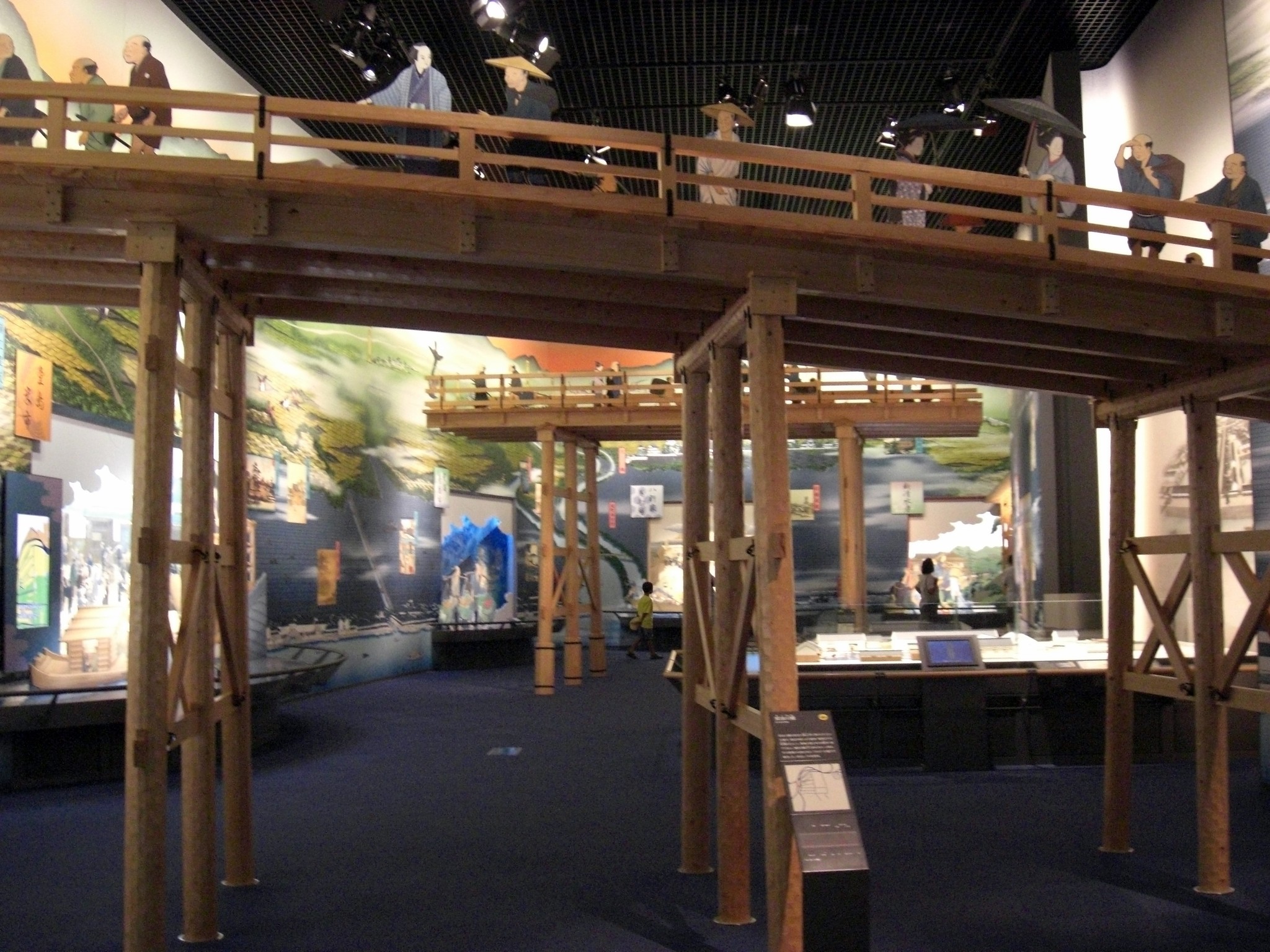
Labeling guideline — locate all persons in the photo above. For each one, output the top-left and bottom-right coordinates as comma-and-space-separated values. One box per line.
0, 33, 44, 148
478, 56, 553, 187
1115, 133, 1174, 259
1183, 153, 1269, 275
356, 42, 452, 174
112, 34, 172, 156
589, 176, 617, 194
624, 581, 663, 661
1185, 252, 1205, 266
659, 377, 674, 407
884, 127, 932, 230
70, 57, 116, 151
916, 558, 940, 622
695, 103, 756, 208
509, 365, 522, 401
475, 367, 488, 407
1003, 553, 1016, 608
1012, 127, 1079, 243
592, 361, 622, 407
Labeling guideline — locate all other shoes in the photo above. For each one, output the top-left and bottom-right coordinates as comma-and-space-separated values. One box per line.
626, 652, 640, 659
649, 653, 664, 660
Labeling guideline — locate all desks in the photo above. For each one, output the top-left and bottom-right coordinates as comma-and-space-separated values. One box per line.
662, 640, 1260, 774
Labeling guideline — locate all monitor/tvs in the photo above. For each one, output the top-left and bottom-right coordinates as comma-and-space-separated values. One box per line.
922, 636, 979, 666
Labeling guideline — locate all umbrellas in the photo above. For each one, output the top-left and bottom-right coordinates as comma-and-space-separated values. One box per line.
880, 111, 989, 192
981, 95, 1082, 177
